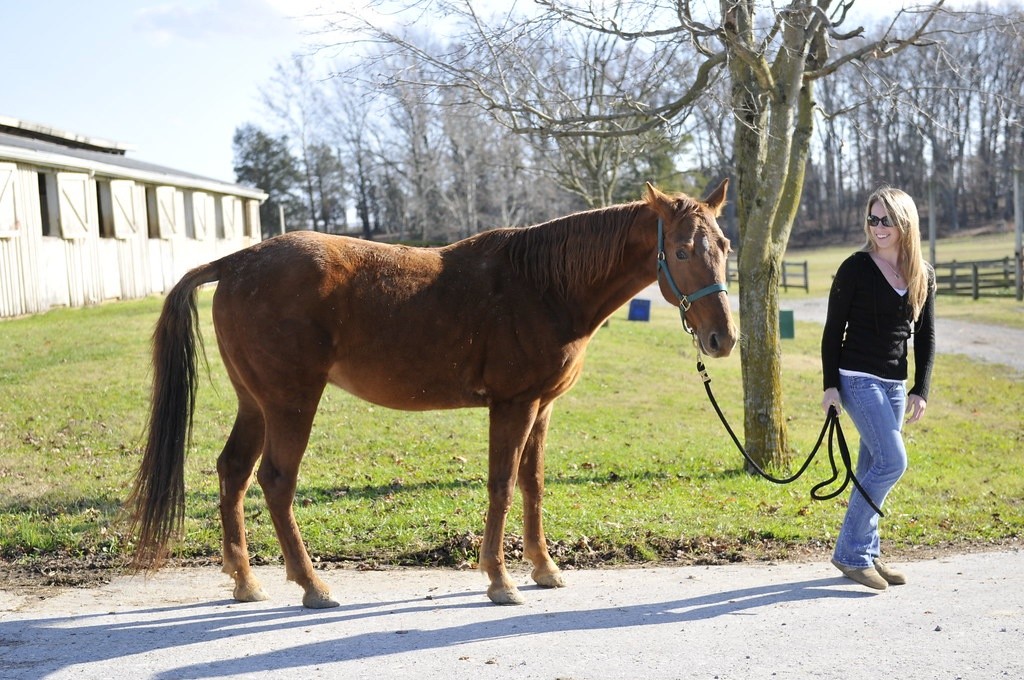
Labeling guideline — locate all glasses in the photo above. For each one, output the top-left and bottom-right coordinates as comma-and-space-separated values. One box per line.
866, 214, 898, 229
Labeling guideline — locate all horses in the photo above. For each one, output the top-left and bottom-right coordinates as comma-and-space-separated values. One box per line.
112, 176, 740, 611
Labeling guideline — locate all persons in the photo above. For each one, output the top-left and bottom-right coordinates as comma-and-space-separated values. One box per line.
820, 185, 937, 590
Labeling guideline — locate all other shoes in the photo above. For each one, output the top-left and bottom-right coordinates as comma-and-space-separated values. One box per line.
870, 558, 905, 586
830, 558, 889, 590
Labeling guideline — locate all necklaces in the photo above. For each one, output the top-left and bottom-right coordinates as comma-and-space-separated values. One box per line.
878, 253, 903, 280
